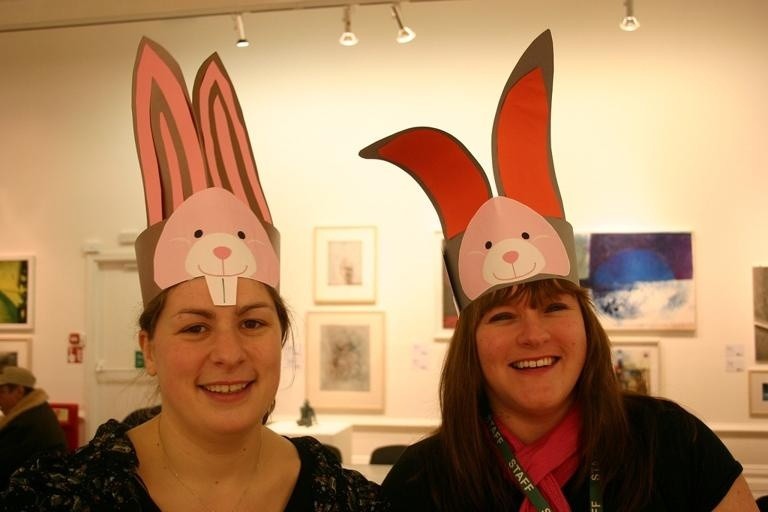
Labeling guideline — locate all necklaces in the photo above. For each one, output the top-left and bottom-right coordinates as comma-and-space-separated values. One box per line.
157, 413, 264, 511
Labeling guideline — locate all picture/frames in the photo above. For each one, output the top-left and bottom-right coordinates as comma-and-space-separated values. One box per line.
601, 340, 663, 396
745, 366, 767, 421
302, 309, 389, 418
310, 224, 380, 306
0, 335, 34, 381
0, 248, 37, 334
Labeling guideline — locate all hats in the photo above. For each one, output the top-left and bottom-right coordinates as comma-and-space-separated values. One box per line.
0, 367, 36, 388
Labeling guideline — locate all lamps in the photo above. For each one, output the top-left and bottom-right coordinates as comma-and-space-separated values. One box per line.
223, 3, 418, 54
618, 0, 639, 36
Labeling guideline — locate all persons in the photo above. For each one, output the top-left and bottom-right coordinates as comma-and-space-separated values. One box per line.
379, 271, 760, 512
0, 269, 379, 511
1, 365, 68, 491
